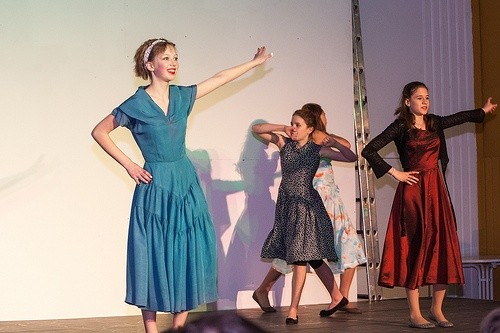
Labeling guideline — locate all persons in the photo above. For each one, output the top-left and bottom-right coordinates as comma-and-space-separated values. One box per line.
90, 37, 274, 333
251, 103, 370, 314
360, 81, 498, 329
251, 109, 358, 324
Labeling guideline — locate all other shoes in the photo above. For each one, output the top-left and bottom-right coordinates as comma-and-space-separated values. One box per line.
285, 310, 299, 325
319, 296, 349, 317
252, 289, 277, 313
408, 319, 435, 329
427, 310, 453, 328
339, 306, 363, 314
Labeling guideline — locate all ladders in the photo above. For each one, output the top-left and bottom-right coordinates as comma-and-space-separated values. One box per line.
351, 0, 383, 303
